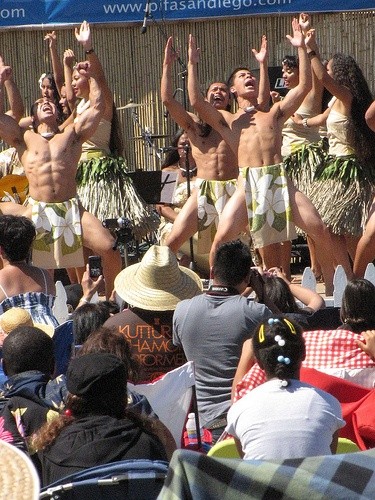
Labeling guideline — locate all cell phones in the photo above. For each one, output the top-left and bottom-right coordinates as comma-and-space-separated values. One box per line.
88, 255, 101, 277
139, 0, 150, 34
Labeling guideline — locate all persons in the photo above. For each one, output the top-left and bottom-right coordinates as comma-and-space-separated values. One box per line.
341, 277, 375, 333
155, 130, 218, 278
171, 239, 274, 451
43, 20, 124, 301
216, 317, 346, 461
0, 305, 55, 386
60, 329, 179, 463
0, 12, 375, 500
113, 244, 205, 338
0, 439, 42, 500
0, 325, 60, 460
38, 355, 170, 488
180, 16, 312, 285
303, 30, 375, 295
160, 34, 237, 274
0, 61, 103, 326
72, 302, 110, 357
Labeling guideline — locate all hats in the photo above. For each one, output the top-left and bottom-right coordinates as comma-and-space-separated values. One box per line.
66, 352, 129, 398
0, 440, 40, 500
0, 307, 56, 348
113, 245, 204, 311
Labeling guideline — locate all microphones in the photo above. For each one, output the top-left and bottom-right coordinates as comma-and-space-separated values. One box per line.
164, 90, 177, 117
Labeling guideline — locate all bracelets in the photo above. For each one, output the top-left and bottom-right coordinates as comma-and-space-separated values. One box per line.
80, 298, 90, 304
307, 49, 316, 58
86, 48, 94, 54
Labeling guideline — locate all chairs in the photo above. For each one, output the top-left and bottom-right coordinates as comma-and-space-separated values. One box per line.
127, 360, 203, 453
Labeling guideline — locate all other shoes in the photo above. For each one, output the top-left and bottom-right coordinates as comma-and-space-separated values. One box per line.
290, 271, 326, 283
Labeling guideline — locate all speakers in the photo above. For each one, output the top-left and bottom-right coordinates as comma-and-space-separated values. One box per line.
232, 66, 288, 113
129, 169, 161, 204
